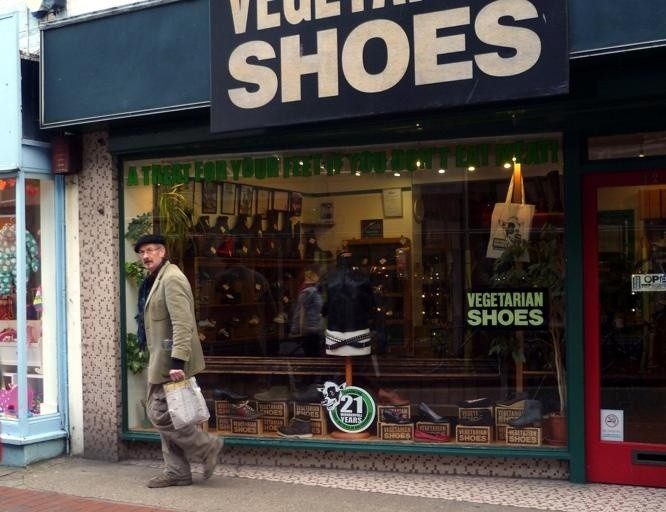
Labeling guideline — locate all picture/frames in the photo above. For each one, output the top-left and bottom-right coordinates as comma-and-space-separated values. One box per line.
381, 187, 404, 219
201, 178, 303, 217
413, 196, 425, 225
318, 199, 337, 226
360, 219, 384, 241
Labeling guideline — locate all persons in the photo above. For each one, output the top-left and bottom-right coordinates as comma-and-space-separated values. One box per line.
133, 233, 223, 488
209, 249, 375, 387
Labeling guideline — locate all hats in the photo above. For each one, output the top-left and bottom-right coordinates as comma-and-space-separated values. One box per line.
135, 235, 165, 253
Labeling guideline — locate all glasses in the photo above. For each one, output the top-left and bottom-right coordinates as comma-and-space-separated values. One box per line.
138, 247, 161, 257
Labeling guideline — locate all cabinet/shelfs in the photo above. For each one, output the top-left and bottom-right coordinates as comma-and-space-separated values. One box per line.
342, 235, 412, 344
185, 255, 319, 350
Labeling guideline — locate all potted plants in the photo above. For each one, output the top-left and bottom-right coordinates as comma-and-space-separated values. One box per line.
487, 217, 568, 447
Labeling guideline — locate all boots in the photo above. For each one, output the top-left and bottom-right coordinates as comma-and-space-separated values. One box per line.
190, 209, 332, 259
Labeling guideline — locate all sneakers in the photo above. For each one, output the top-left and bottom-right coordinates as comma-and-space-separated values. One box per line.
213, 383, 329, 439
147, 472, 192, 488
203, 438, 224, 480
197, 317, 217, 330
272, 312, 291, 324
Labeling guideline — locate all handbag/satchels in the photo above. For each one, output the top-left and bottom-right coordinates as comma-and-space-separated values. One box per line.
486, 202, 537, 263
162, 377, 211, 431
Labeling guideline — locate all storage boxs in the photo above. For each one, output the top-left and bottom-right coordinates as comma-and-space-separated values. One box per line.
212, 400, 329, 439
375, 401, 543, 446
638, 188, 666, 220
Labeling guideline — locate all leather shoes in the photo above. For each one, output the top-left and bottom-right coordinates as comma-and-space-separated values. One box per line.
377, 387, 543, 443
